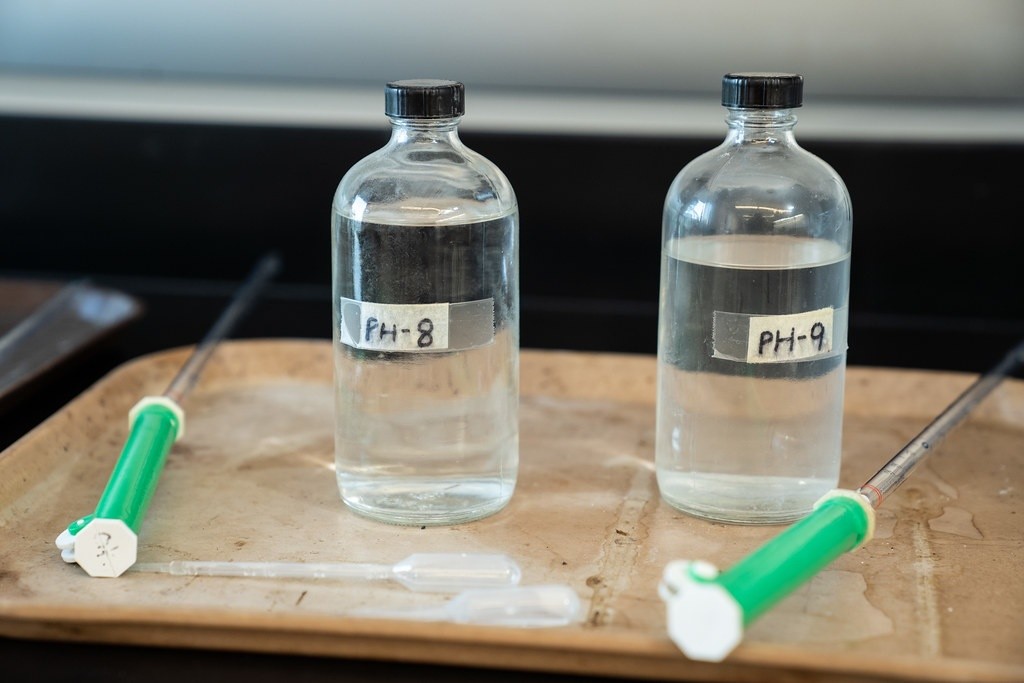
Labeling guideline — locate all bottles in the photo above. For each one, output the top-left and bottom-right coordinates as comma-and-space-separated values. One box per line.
330, 79, 521, 527
655, 71, 852, 525
